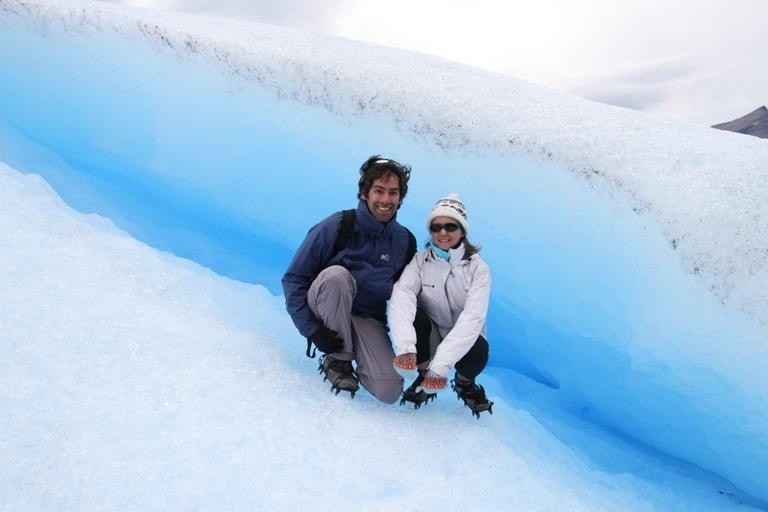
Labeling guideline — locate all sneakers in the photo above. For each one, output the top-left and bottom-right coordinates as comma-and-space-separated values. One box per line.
321, 354, 358, 393
403, 371, 440, 404
451, 371, 490, 413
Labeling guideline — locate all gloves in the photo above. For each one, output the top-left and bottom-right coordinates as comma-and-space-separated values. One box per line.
390, 352, 419, 382
310, 324, 345, 354
421, 371, 448, 395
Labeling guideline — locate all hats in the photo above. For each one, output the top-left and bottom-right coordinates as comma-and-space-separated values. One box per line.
426, 192, 469, 234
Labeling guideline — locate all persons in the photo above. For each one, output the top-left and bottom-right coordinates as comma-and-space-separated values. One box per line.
279, 153, 417, 404
386, 191, 493, 414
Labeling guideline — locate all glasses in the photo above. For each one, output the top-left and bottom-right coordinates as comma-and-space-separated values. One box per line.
364, 157, 405, 175
430, 222, 460, 233
